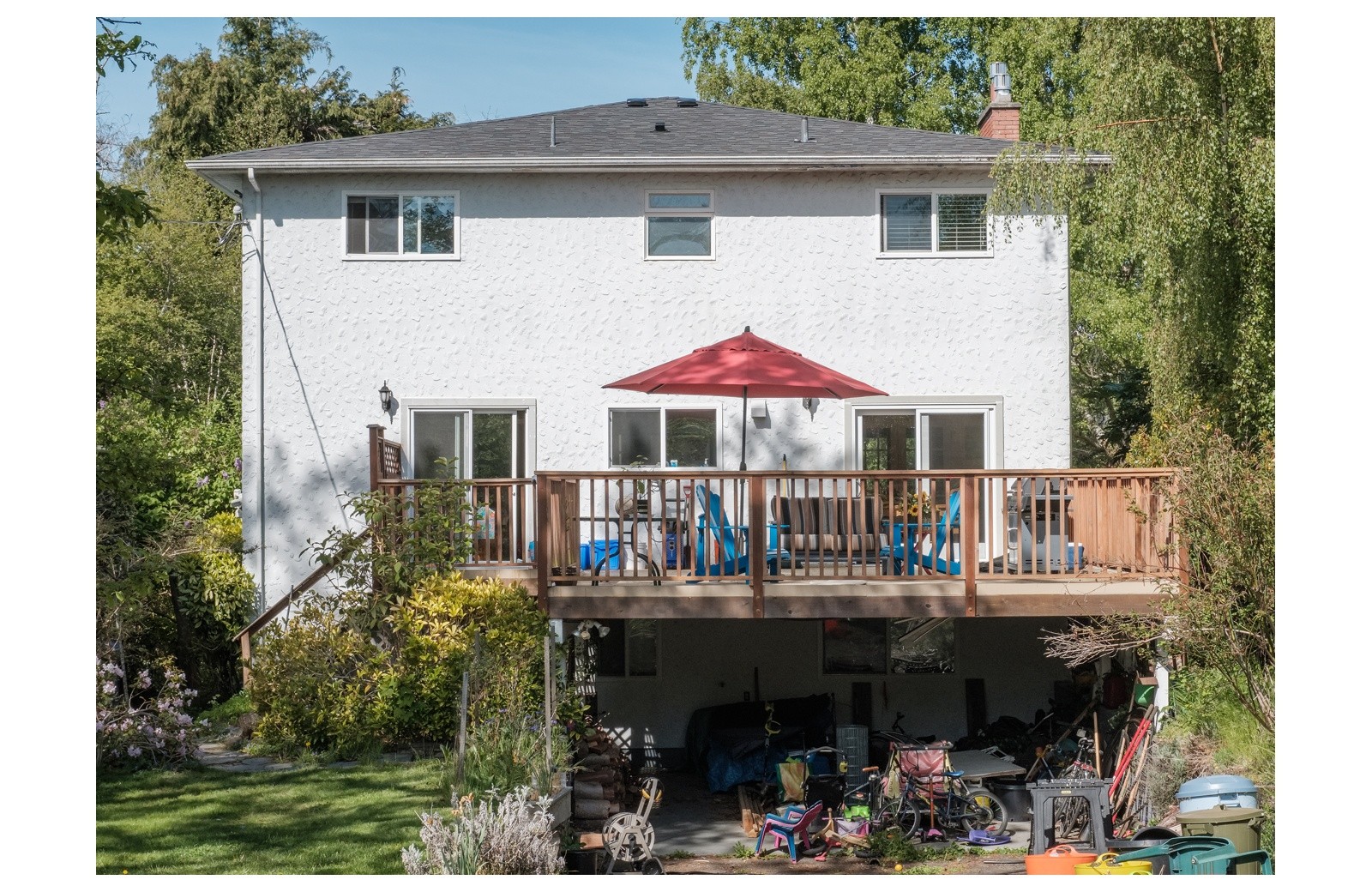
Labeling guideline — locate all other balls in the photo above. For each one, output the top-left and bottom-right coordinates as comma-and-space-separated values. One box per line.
615, 495, 634, 518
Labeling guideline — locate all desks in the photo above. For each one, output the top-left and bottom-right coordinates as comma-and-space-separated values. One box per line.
573, 515, 676, 586
900, 530, 932, 575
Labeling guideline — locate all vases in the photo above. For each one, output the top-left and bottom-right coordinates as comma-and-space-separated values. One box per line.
907, 514, 918, 523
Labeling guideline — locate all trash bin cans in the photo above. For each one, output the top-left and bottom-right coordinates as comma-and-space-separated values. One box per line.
1176, 803, 1266, 874
1176, 775, 1259, 813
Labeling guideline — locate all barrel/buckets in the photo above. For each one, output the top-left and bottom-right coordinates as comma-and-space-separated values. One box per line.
623, 543, 646, 570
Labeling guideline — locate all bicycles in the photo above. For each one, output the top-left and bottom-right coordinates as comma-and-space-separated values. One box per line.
800, 746, 896, 835
874, 741, 1008, 843
1046, 736, 1104, 840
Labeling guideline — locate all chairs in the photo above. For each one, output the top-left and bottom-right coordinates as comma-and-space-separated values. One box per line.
755, 799, 823, 863
1111, 835, 1273, 875
687, 485, 790, 585
880, 490, 960, 574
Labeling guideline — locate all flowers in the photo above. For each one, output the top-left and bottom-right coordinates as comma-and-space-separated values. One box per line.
899, 492, 941, 519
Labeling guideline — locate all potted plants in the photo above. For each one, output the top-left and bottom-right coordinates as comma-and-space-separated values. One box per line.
617, 455, 658, 516
560, 818, 598, 875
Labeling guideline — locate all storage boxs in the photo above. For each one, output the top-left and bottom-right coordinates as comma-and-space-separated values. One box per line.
1068, 543, 1083, 569
588, 540, 620, 570
580, 545, 589, 570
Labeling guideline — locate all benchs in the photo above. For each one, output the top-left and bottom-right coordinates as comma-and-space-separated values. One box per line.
771, 495, 887, 568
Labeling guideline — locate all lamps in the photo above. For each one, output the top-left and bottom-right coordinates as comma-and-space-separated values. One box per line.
572, 619, 610, 640
803, 398, 812, 410
378, 380, 391, 413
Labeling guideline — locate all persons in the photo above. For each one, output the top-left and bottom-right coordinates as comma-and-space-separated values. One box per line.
601, 326, 889, 558
